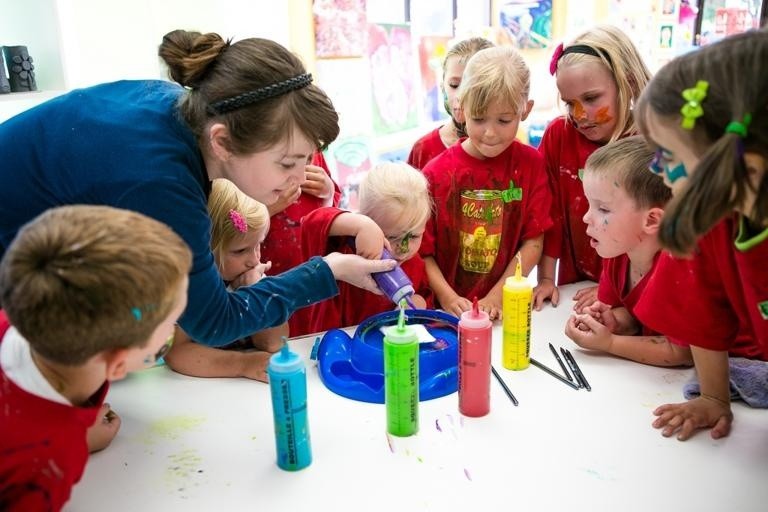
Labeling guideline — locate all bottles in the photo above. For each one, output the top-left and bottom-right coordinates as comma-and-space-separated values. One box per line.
503, 264, 531, 370
457, 298, 494, 417
382, 309, 420, 436
264, 338, 312, 471
373, 247, 420, 311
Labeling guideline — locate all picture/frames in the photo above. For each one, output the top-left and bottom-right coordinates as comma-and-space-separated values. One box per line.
691, 0, 768, 48
489, 0, 557, 53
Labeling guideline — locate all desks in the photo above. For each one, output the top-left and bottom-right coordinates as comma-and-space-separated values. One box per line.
59, 279, 768, 511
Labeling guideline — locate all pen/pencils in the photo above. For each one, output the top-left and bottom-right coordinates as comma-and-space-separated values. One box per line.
492, 366, 520, 408
530, 342, 591, 393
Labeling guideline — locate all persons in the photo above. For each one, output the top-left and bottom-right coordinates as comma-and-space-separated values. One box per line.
0, 29, 397, 347
632, 29, 768, 441
565, 135, 694, 367
417, 47, 553, 324
260, 144, 342, 338
300, 160, 433, 334
407, 38, 495, 172
1, 204, 193, 512
532, 27, 653, 312
162, 178, 290, 383
